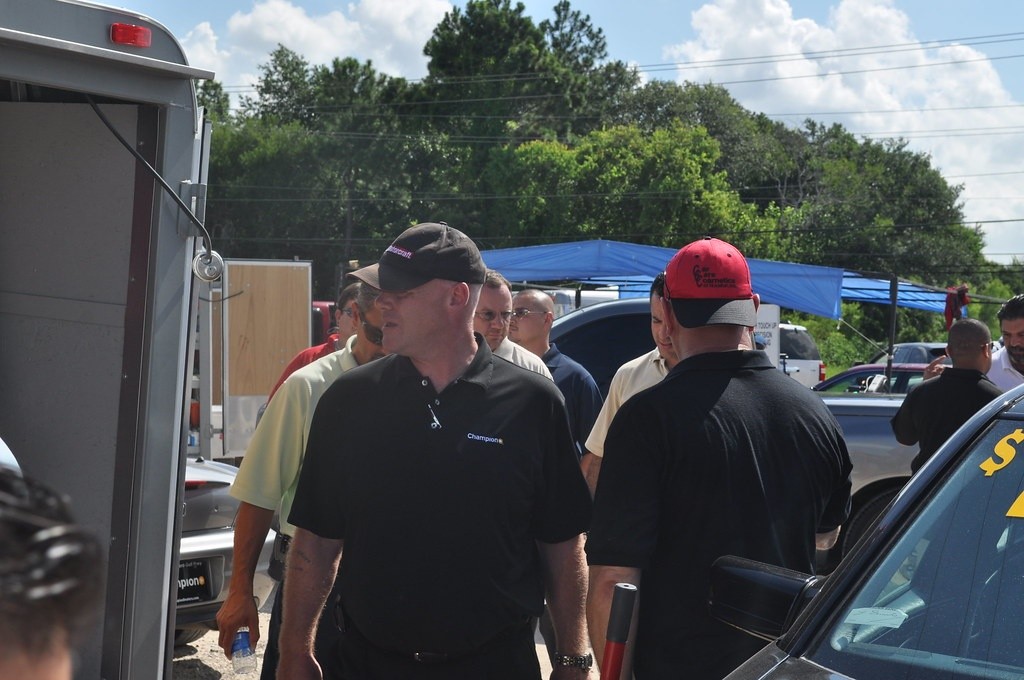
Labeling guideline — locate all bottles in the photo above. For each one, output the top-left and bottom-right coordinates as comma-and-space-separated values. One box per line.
230, 626, 257, 674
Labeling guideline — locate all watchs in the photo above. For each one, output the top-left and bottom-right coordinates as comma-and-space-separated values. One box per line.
550, 649, 593, 672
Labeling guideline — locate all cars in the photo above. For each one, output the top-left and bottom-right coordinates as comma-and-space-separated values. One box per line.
172, 457, 279, 649
853, 341, 955, 394
702, 383, 1024, 680
775, 324, 827, 391
808, 362, 952, 498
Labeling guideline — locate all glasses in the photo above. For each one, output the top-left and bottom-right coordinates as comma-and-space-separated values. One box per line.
511, 310, 547, 319
358, 312, 383, 347
475, 311, 510, 322
341, 308, 352, 317
980, 343, 994, 350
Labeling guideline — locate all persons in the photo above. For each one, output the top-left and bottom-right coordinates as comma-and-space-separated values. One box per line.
891, 318, 1001, 477
586, 244, 851, 680
276, 222, 600, 680
216, 262, 760, 680
986, 293, 1024, 394
0, 436, 109, 680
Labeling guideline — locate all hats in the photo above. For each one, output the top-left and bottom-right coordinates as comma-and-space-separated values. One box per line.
345, 221, 486, 292
754, 336, 767, 348
664, 236, 757, 328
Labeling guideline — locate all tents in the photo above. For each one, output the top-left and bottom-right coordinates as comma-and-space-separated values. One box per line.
483, 240, 1005, 366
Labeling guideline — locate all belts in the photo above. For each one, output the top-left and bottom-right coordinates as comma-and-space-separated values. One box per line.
397, 625, 514, 665
